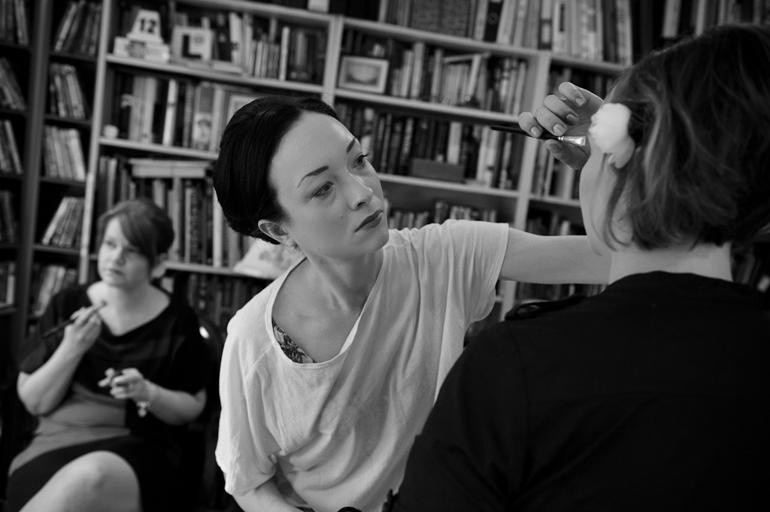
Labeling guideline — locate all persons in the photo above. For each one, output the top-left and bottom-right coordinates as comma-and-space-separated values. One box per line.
7, 197, 208, 512
213, 80, 605, 511
398, 23, 770, 512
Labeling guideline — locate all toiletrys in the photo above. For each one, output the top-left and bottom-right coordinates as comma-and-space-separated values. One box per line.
103, 367, 125, 389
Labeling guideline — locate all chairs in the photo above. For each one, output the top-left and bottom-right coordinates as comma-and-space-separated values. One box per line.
143, 316, 226, 512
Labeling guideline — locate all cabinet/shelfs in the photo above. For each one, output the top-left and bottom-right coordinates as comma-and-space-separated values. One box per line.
2, 0, 97, 467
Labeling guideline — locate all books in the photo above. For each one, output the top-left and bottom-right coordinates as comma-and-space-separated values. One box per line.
1, 2, 767, 337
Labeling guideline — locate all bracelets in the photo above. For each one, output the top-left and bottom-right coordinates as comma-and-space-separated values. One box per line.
135, 381, 155, 407
